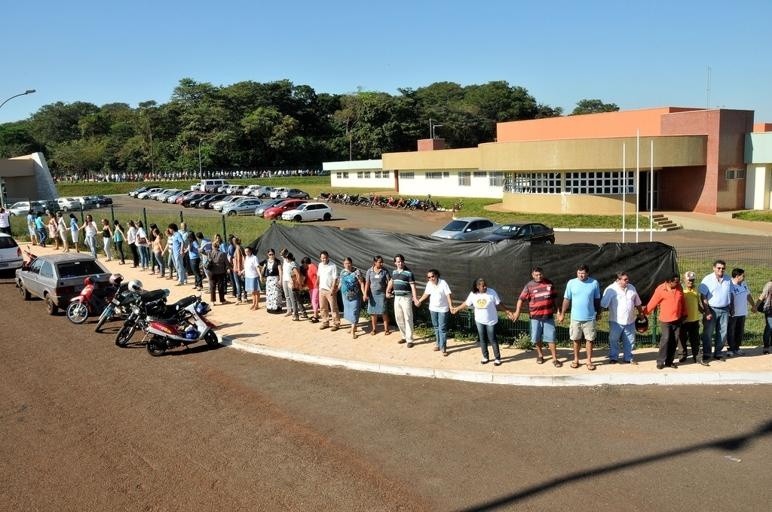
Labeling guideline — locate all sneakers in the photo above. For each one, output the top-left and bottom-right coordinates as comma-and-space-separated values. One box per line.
434, 347, 439, 351
442, 352, 448, 356
331, 326, 339, 331
320, 325, 328, 329
481, 358, 489, 364
609, 360, 617, 364
407, 343, 413, 348
494, 359, 502, 366
727, 351, 734, 357
735, 350, 745, 356
657, 356, 726, 369
629, 359, 638, 365
399, 339, 406, 344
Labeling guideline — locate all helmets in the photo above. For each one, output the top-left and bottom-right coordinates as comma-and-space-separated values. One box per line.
196, 301, 211, 315
185, 327, 197, 339
109, 273, 123, 287
127, 279, 143, 294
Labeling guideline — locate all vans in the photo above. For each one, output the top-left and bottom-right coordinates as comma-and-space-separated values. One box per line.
200, 179, 228, 193
264, 199, 310, 220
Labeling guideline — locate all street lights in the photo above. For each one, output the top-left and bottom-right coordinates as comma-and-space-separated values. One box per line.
429, 118, 439, 138
0, 88, 36, 109
199, 137, 210, 179
432, 124, 444, 138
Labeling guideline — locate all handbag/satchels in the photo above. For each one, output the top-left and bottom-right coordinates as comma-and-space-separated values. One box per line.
757, 292, 769, 312
635, 316, 649, 333
137, 233, 146, 244
347, 284, 363, 301
263, 260, 269, 277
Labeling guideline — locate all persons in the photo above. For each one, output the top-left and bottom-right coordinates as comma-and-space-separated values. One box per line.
452, 279, 513, 366
642, 271, 705, 369
0, 208, 13, 236
162, 222, 262, 310
386, 255, 421, 348
751, 282, 771, 355
597, 270, 644, 364
699, 260, 757, 363
314, 251, 365, 338
363, 256, 391, 335
27, 209, 47, 246
45, 209, 69, 252
126, 220, 165, 278
258, 248, 320, 323
559, 263, 601, 370
513, 267, 562, 368
56, 166, 331, 183
419, 269, 455, 356
67, 214, 80, 253
79, 214, 127, 264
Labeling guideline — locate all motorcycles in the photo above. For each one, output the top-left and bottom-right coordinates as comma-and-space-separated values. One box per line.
66, 275, 147, 324
139, 294, 219, 357
93, 278, 170, 334
114, 292, 203, 346
317, 191, 445, 213
23, 249, 37, 268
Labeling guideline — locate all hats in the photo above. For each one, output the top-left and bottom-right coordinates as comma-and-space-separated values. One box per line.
683, 271, 696, 282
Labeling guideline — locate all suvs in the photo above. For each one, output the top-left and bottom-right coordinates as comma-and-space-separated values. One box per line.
36, 199, 59, 213
7, 201, 44, 218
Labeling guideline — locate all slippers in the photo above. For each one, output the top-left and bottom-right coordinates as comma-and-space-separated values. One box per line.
570, 361, 579, 368
537, 354, 543, 364
553, 360, 562, 367
586, 364, 596, 370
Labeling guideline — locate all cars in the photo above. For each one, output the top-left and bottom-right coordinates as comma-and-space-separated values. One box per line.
208, 184, 310, 200
479, 220, 556, 248
129, 186, 262, 217
254, 199, 285, 217
281, 202, 333, 223
431, 217, 503, 242
0, 231, 23, 270
190, 183, 200, 191
13, 253, 112, 314
54, 194, 112, 212
